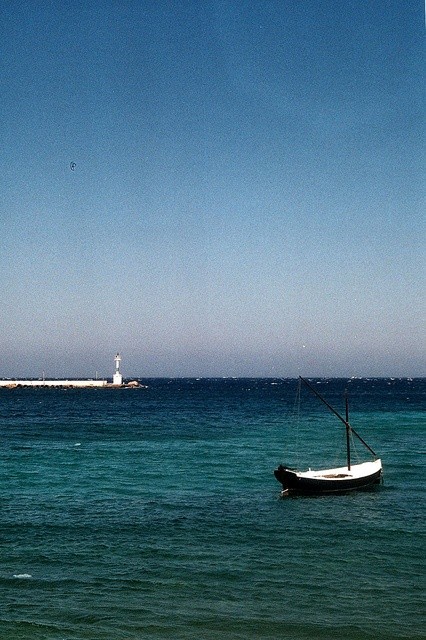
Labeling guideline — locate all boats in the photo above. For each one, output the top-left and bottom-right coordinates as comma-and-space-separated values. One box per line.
275, 375, 382, 496
